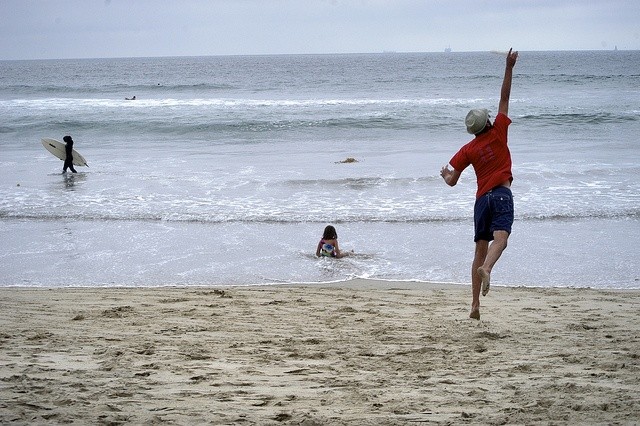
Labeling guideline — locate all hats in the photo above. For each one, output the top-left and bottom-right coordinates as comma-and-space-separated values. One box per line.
465, 107, 488, 134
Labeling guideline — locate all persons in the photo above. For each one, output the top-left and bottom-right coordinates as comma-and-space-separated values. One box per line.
63, 136, 77, 173
440, 48, 519, 319
316, 225, 348, 259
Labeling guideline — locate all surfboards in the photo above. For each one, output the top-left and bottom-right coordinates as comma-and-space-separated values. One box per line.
41, 138, 87, 166
